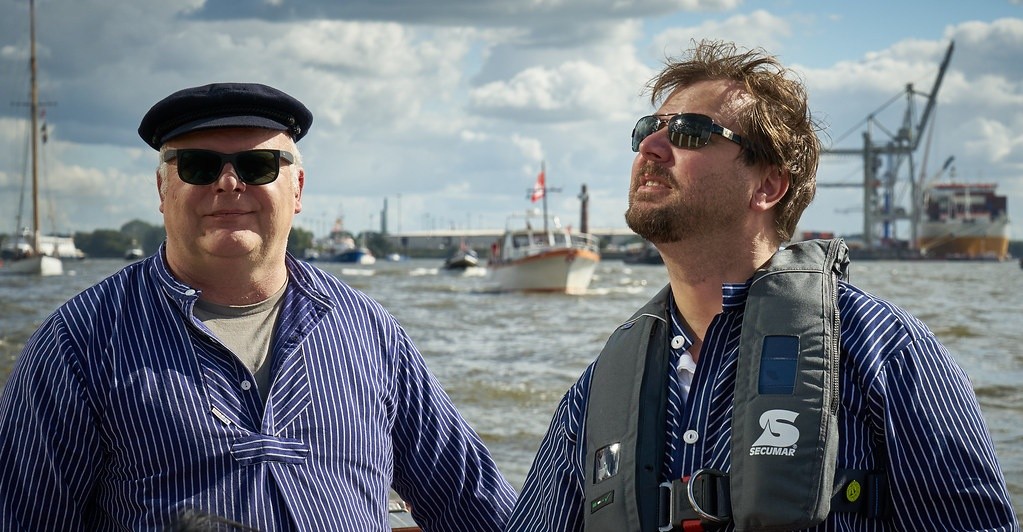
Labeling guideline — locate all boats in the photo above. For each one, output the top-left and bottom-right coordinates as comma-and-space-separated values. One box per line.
444, 241, 478, 268
305, 217, 404, 265
915, 168, 1011, 261
1, 225, 86, 260
125, 238, 143, 260
619, 238, 661, 264
485, 158, 601, 294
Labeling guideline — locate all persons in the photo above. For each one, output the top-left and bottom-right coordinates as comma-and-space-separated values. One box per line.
504, 38, 1019, 532
0, 82, 518, 532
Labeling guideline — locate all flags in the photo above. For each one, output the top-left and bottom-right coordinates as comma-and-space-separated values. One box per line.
532, 172, 545, 201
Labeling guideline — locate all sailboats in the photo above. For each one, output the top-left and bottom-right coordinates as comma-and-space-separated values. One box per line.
2, 0, 64, 277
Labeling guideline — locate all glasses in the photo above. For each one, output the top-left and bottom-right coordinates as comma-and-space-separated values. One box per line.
163, 147, 294, 185
630, 113, 745, 152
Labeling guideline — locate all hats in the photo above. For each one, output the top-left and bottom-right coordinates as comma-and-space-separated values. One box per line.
138, 82, 313, 152
